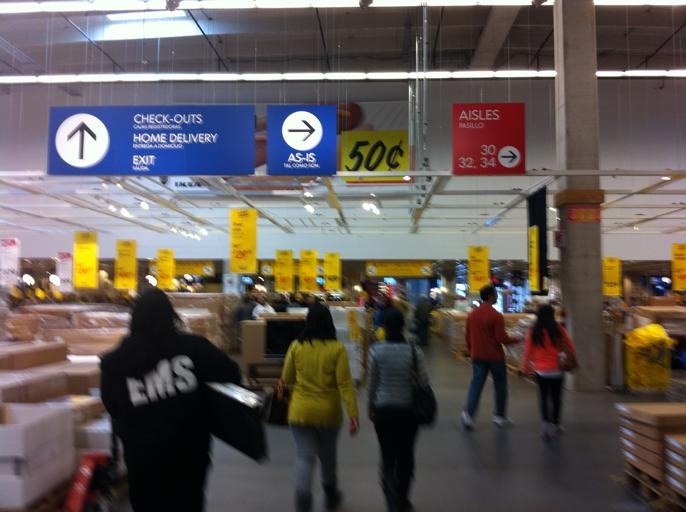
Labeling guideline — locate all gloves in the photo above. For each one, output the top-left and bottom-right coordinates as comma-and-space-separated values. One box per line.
349, 416, 360, 435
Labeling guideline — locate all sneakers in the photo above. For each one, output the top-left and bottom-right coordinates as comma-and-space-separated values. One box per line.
459, 410, 479, 429
539, 421, 568, 439
491, 415, 515, 427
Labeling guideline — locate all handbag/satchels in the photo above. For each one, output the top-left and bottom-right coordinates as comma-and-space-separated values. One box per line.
410, 381, 439, 428
262, 379, 293, 427
557, 349, 579, 373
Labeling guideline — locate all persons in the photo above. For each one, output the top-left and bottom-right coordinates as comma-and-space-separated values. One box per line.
236, 285, 346, 322
278, 305, 357, 509
366, 311, 432, 510
457, 285, 523, 430
416, 294, 431, 344
99, 288, 238, 512
521, 304, 579, 440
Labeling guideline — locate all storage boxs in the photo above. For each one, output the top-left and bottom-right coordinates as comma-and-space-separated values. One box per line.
439, 297, 685, 389
167, 292, 370, 401
0, 291, 128, 509
614, 400, 685, 501
